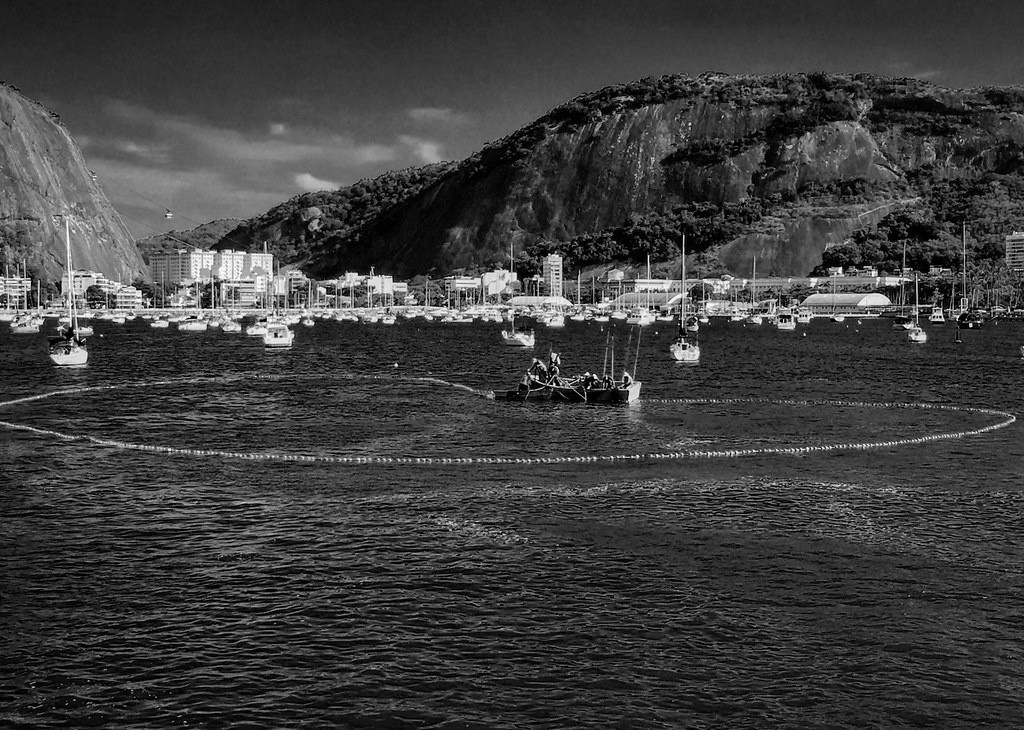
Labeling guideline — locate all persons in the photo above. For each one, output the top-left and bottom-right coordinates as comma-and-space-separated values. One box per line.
528, 351, 561, 385
583, 371, 634, 389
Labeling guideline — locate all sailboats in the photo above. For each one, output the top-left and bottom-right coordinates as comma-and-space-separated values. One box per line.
890, 237, 1024, 343
0, 233, 811, 364
47, 219, 88, 364
830, 276, 845, 322
502, 242, 534, 346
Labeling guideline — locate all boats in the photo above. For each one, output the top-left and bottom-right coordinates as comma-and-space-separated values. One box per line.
519, 324, 642, 405
